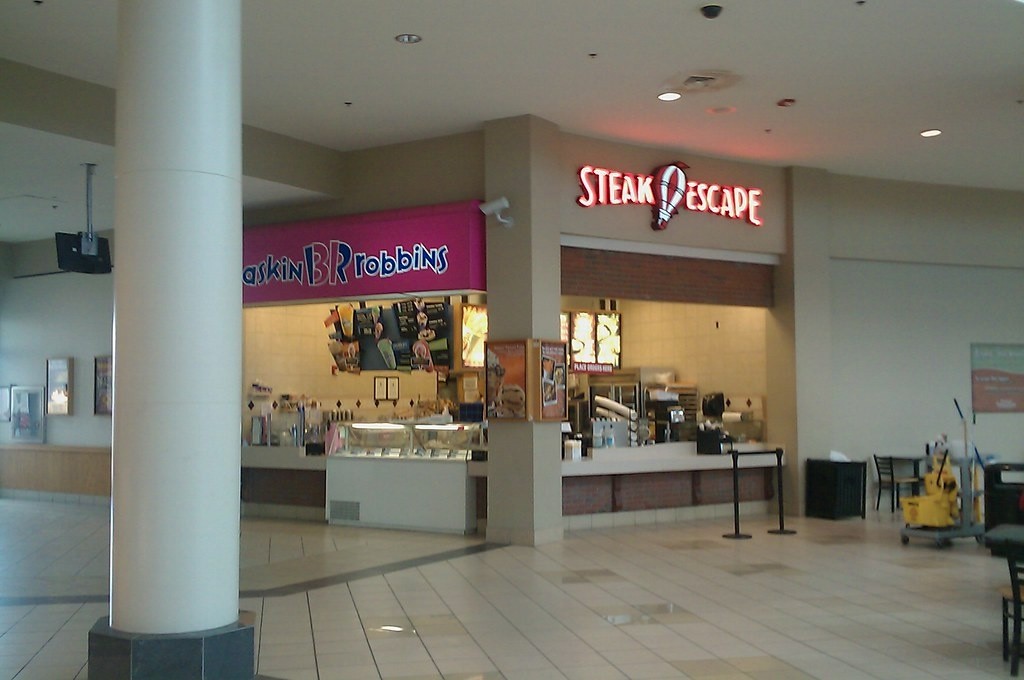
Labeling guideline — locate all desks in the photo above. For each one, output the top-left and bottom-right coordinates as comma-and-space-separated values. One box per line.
983, 524, 1024, 559
878, 453, 928, 496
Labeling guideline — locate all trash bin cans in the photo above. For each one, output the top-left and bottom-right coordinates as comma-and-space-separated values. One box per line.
805, 457, 867, 520
982, 461, 1024, 549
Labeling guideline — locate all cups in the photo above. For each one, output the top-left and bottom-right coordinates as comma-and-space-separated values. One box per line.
338, 306, 353, 337
378, 339, 396, 368
595, 395, 637, 441
413, 340, 433, 370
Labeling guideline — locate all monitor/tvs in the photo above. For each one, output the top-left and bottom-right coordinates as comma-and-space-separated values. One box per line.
55, 231, 112, 274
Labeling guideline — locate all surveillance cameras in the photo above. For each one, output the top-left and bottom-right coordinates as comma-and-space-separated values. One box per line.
701, 5, 723, 19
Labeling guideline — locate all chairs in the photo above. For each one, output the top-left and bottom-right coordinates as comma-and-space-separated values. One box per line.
873, 454, 921, 514
1001, 537, 1024, 676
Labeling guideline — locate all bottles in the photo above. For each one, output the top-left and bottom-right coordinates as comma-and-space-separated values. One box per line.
605, 423, 616, 448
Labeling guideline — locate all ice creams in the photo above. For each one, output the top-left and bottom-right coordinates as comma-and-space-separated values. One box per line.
372, 305, 384, 343
